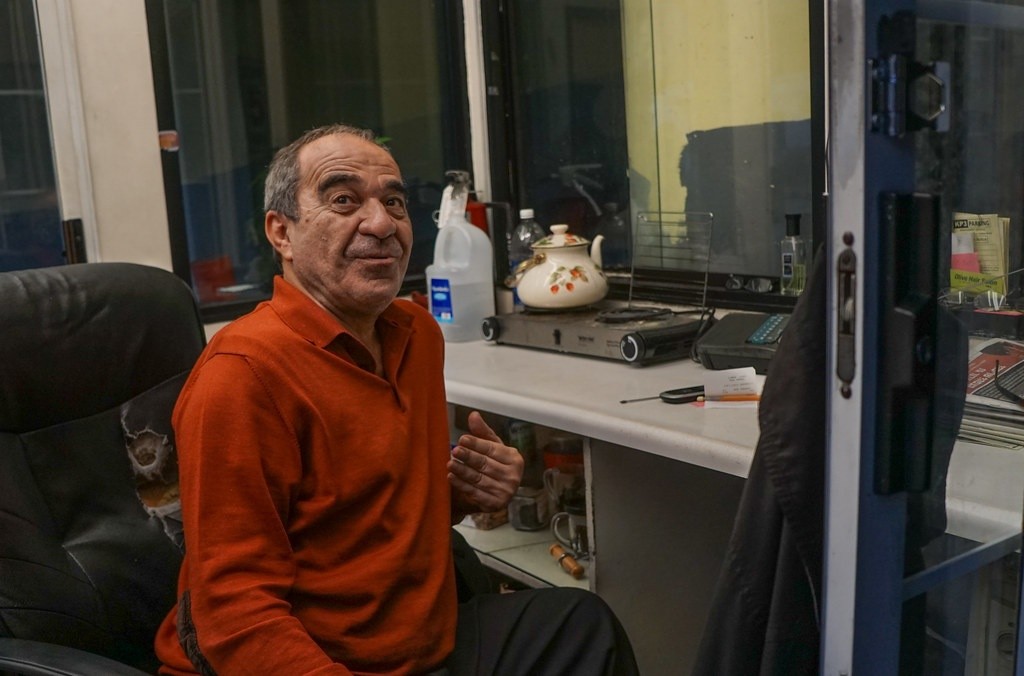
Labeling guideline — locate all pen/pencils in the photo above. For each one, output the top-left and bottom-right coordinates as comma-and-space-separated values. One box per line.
696, 393, 760, 402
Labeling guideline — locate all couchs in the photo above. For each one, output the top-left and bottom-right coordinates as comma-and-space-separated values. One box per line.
0, 261, 494, 676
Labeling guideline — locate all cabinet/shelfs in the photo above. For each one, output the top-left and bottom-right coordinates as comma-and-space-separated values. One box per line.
449, 400, 743, 676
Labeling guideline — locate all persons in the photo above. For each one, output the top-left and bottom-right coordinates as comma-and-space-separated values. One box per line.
154, 125, 640, 675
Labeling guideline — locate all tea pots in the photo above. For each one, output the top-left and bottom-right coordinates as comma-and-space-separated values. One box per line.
514, 224, 609, 308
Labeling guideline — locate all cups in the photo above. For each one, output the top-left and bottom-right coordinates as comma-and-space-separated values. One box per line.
543, 465, 586, 509
508, 486, 552, 530
551, 497, 589, 554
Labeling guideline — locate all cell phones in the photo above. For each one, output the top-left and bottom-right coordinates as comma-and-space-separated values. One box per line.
660, 384, 705, 405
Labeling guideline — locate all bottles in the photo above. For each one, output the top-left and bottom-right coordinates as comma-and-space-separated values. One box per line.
512, 207, 546, 311
425, 213, 495, 343
